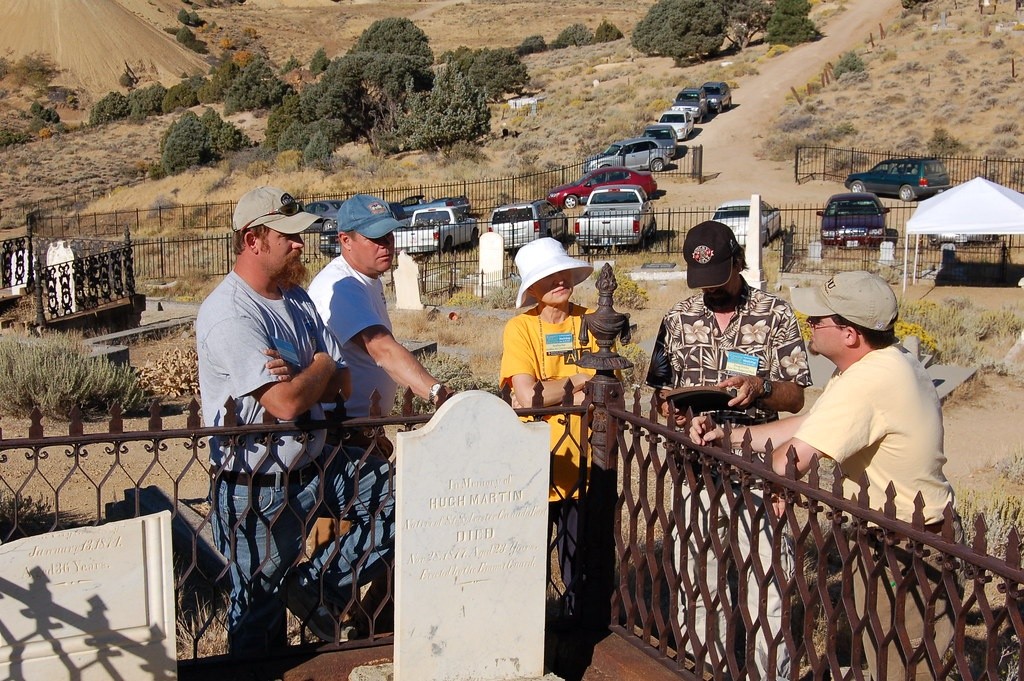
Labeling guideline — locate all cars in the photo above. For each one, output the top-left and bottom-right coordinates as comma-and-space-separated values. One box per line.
927, 234, 998, 245
639, 125, 677, 157
711, 199, 783, 247
818, 192, 891, 246
844, 158, 956, 200
544, 165, 658, 209
300, 200, 344, 231
656, 109, 694, 140
318, 202, 407, 259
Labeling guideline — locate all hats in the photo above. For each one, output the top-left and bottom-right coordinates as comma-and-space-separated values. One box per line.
683, 221, 741, 289
514, 237, 594, 310
790, 271, 899, 332
232, 186, 324, 235
336, 193, 402, 239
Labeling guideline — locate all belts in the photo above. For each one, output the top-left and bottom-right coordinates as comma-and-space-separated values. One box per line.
211, 456, 323, 487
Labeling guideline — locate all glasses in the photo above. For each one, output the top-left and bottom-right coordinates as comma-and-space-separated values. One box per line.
240, 201, 305, 231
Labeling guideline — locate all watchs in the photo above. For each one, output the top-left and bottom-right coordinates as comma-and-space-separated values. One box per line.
757, 376, 772, 400
429, 383, 444, 406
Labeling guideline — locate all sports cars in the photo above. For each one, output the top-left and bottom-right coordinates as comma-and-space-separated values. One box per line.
399, 194, 471, 215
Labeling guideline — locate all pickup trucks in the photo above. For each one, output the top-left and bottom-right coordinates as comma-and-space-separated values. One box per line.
393, 206, 479, 256
574, 183, 657, 253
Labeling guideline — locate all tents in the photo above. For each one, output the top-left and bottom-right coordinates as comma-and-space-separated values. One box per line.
903, 176, 1024, 292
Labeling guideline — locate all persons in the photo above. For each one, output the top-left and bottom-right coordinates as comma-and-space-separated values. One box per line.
308, 194, 453, 641
689, 270, 965, 681
646, 222, 813, 681
195, 186, 396, 654
499, 238, 620, 631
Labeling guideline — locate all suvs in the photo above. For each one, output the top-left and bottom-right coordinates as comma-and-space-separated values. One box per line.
700, 81, 733, 112
488, 200, 569, 252
581, 135, 671, 174
671, 87, 709, 123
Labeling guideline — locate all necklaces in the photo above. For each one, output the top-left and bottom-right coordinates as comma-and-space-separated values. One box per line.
537, 305, 579, 381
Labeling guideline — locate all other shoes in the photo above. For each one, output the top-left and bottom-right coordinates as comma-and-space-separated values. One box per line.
281, 577, 341, 642
